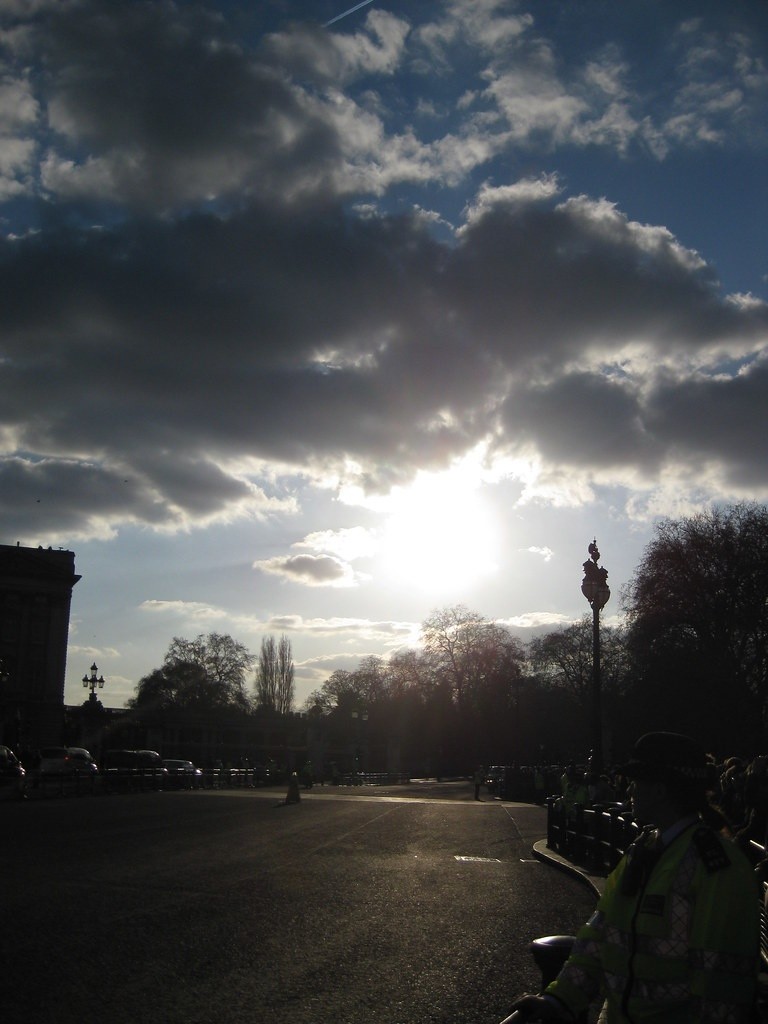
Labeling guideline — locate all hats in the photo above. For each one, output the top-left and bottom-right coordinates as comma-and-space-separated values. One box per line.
618, 731, 722, 788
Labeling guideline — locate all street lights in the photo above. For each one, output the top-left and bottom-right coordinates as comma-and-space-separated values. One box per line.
582, 535, 611, 808
83, 662, 105, 761
509, 666, 525, 766
350, 702, 369, 771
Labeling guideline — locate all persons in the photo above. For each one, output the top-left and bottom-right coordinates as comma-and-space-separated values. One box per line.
510, 731, 759, 1023
207, 751, 345, 789
21, 745, 32, 778
472, 754, 768, 894
31, 750, 42, 788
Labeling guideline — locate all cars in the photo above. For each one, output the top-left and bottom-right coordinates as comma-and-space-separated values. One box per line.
485, 765, 505, 791
1, 746, 412, 800
